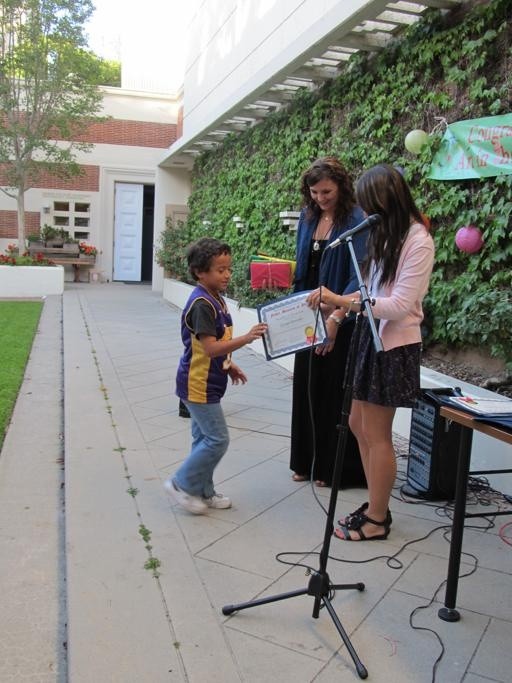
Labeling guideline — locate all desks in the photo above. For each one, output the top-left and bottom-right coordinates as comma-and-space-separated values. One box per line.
386, 365, 512, 597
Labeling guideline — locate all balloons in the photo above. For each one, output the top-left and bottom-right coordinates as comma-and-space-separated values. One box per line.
404, 129, 427, 155
456, 225, 484, 254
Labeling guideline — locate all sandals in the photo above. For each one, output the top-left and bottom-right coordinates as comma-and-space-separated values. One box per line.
337, 501, 394, 525
334, 509, 395, 542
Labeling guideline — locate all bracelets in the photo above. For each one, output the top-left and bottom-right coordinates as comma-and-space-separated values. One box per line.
329, 315, 342, 325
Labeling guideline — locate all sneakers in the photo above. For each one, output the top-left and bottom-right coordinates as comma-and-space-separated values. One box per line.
165, 478, 207, 515
203, 488, 233, 511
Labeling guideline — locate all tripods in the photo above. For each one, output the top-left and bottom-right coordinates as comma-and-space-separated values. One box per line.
211, 250, 386, 680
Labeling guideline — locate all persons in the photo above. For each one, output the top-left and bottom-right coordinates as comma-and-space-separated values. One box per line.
304, 163, 435, 543
164, 236, 267, 515
259, 155, 370, 489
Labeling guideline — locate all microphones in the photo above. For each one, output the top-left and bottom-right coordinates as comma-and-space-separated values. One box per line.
327, 211, 383, 249
